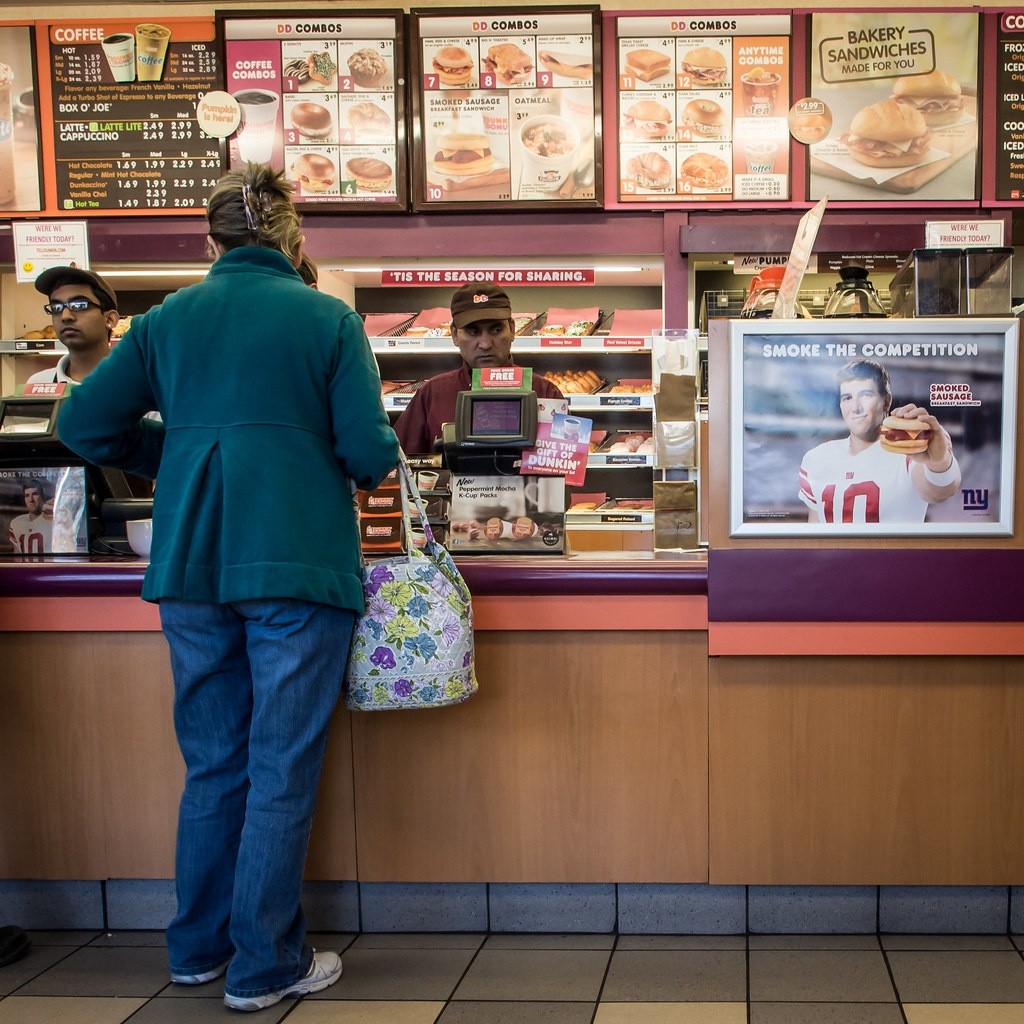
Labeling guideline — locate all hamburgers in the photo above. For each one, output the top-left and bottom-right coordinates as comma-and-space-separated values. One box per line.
623, 47, 728, 188
433, 135, 494, 176
794, 113, 827, 139
893, 70, 965, 124
877, 416, 932, 455
839, 100, 930, 169
433, 44, 532, 85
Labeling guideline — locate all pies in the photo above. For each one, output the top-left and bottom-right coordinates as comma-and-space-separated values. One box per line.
538, 50, 593, 79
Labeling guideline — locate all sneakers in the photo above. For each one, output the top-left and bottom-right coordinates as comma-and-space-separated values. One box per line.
169, 958, 231, 984
224, 947, 342, 1011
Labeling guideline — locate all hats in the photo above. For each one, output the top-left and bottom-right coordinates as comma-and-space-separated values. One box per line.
34, 266, 117, 310
450, 282, 512, 328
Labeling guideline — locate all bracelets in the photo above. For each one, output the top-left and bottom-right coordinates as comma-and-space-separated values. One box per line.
924, 454, 960, 487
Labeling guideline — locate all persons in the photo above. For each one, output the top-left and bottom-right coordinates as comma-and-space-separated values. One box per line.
393, 281, 572, 512
27, 266, 163, 492
56, 162, 402, 1010
9, 480, 77, 562
798, 357, 962, 523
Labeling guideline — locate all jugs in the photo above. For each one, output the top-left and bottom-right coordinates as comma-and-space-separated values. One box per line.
824, 267, 890, 318
740, 266, 804, 318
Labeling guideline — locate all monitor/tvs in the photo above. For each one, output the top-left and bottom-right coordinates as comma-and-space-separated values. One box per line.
440, 423, 572, 513
0, 397, 133, 497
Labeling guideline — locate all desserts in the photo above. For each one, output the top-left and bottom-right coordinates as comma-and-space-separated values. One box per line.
287, 48, 392, 191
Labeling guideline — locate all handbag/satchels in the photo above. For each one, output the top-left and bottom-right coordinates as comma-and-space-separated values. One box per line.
342, 444, 479, 711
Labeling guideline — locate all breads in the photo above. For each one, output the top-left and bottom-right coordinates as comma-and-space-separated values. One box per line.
379, 316, 655, 514
22, 325, 56, 341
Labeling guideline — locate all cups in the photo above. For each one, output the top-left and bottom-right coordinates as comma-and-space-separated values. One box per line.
101, 33, 135, 82
232, 88, 280, 164
135, 24, 171, 81
126, 519, 153, 558
564, 418, 581, 436
525, 478, 565, 512
741, 73, 781, 118
407, 471, 439, 549
518, 115, 576, 194
743, 145, 776, 174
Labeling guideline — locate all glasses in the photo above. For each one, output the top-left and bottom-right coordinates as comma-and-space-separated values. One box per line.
43, 299, 102, 315
